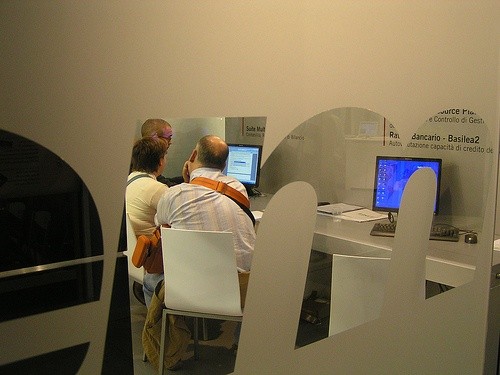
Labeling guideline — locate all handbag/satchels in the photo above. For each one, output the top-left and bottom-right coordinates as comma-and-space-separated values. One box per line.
142, 225, 172, 274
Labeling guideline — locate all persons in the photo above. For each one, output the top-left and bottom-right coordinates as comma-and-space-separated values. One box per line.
125, 132, 170, 238
143, 135, 258, 349
141, 119, 184, 188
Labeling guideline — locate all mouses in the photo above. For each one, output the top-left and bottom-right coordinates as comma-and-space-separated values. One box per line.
465, 234, 478, 244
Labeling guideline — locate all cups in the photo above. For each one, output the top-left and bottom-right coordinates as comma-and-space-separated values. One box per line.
333, 207, 342, 221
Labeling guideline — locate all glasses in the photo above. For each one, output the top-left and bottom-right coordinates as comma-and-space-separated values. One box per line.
157, 135, 172, 142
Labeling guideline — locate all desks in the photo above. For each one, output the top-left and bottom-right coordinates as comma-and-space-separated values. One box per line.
250, 193, 500, 288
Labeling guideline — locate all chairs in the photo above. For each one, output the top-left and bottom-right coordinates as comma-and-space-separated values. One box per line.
126, 214, 244, 375
328, 254, 426, 336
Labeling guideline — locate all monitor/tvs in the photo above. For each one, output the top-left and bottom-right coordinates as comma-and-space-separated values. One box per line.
372, 156, 442, 215
220, 144, 263, 192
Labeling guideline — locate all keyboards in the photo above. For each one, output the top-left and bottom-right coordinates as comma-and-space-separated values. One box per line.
370, 222, 458, 241
